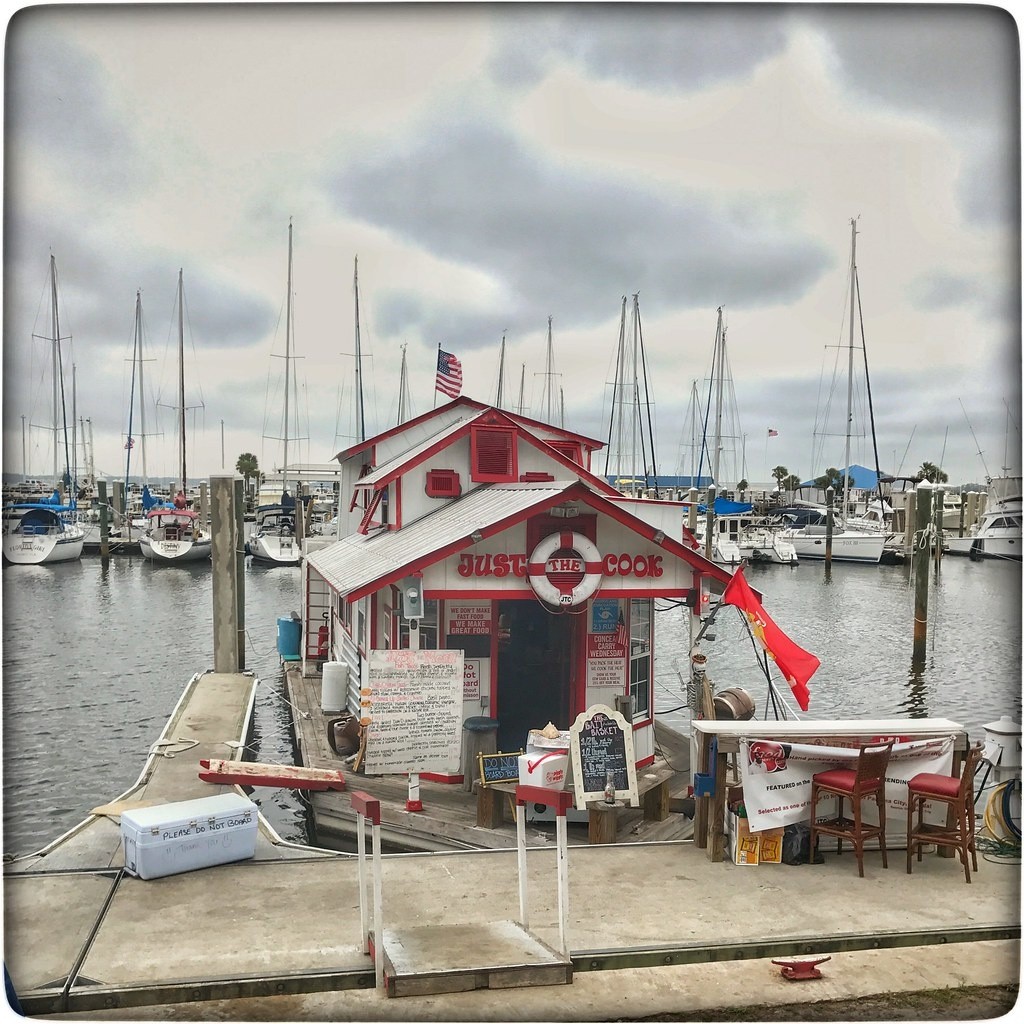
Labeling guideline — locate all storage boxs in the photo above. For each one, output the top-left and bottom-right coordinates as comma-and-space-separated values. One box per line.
726, 787, 783, 867
121, 792, 258, 881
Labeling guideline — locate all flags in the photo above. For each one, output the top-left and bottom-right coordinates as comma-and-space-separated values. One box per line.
720, 568, 821, 712
769, 430, 778, 436
125, 438, 135, 450
436, 350, 462, 399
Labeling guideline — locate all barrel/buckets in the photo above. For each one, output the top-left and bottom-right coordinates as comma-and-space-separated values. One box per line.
332, 717, 360, 755
713, 686, 755, 720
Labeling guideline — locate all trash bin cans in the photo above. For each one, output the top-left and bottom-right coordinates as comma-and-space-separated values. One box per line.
277, 617, 299, 655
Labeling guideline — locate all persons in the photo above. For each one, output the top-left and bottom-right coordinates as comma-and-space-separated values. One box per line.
172, 519, 181, 540
749, 752, 767, 775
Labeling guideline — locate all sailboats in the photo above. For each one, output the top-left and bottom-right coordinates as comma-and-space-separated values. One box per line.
2, 212, 1021, 567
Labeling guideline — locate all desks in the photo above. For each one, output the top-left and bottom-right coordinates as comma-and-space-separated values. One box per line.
691, 718, 966, 862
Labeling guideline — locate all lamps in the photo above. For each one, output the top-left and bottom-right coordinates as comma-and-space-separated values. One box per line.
550, 506, 579, 519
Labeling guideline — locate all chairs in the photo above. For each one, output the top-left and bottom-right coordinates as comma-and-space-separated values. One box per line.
906, 741, 986, 883
809, 737, 895, 877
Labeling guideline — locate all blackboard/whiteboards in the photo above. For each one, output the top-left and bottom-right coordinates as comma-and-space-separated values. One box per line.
476, 751, 524, 785
568, 704, 640, 801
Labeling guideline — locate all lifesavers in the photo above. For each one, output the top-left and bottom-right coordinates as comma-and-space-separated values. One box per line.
529, 533, 602, 607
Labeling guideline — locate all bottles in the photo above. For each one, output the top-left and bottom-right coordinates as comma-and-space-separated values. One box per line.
604, 771, 615, 803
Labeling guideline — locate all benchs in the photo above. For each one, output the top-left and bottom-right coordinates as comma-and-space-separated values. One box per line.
473, 778, 624, 845
587, 768, 675, 844
